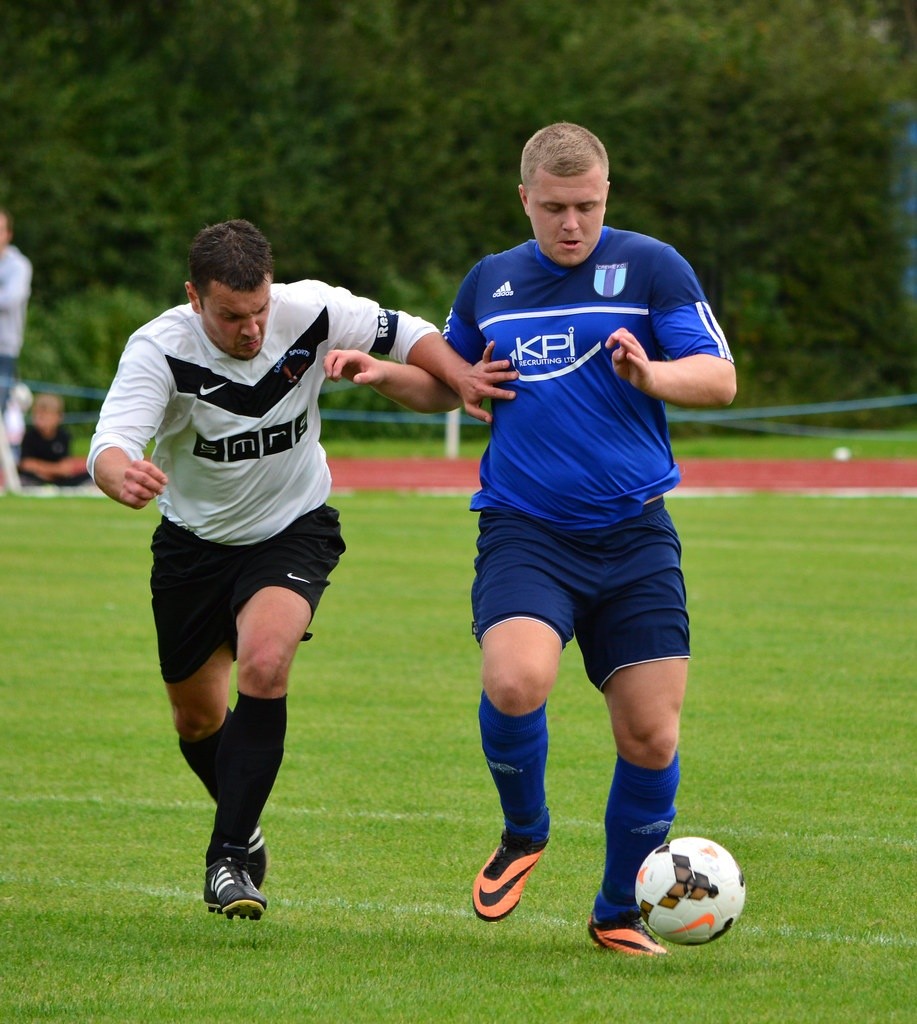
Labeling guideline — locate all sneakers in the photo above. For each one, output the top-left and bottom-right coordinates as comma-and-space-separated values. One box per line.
586, 912, 668, 957
246, 819, 267, 890
202, 857, 268, 921
472, 830, 550, 922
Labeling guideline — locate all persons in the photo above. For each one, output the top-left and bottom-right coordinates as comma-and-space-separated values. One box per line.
84, 219, 521, 920
0, 208, 33, 413
323, 123, 739, 956
15, 391, 94, 489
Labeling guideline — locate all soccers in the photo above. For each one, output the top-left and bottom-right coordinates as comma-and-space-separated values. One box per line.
634, 835, 747, 947
